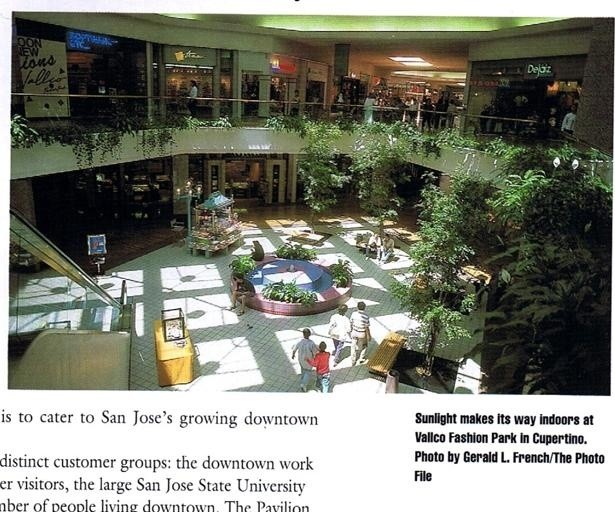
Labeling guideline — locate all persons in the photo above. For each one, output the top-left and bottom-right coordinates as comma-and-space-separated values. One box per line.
479, 102, 504, 134
365, 232, 382, 261
292, 328, 318, 392
380, 234, 395, 264
363, 94, 378, 125
288, 90, 301, 116
328, 304, 352, 367
561, 103, 577, 143
391, 90, 457, 130
349, 302, 371, 366
513, 92, 528, 107
305, 341, 331, 393
188, 80, 198, 118
249, 239, 264, 261
473, 278, 490, 306
228, 273, 256, 316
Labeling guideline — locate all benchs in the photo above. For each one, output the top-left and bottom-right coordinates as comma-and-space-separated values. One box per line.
365, 330, 408, 383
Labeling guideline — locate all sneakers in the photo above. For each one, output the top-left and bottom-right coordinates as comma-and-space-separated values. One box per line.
300, 384, 306, 392
237, 311, 244, 316
225, 304, 235, 311
